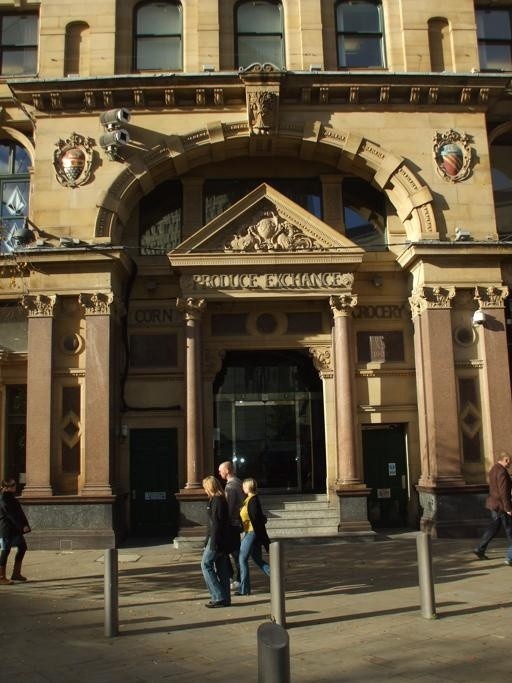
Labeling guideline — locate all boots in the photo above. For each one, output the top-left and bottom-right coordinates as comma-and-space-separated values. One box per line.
11, 561, 26, 580
0, 565, 14, 585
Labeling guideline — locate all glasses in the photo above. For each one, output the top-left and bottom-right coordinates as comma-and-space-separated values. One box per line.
5, 482, 16, 488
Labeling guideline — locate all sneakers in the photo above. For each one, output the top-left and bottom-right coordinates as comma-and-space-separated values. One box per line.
205, 599, 231, 608
233, 590, 250, 596
473, 549, 488, 560
504, 558, 512, 565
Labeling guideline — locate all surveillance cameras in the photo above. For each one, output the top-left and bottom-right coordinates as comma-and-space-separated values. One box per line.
473, 310, 486, 325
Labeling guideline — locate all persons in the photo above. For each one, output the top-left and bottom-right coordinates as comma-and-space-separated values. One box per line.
235, 475, 270, 596
1, 475, 32, 584
199, 475, 234, 609
473, 450, 512, 568
217, 461, 246, 582
